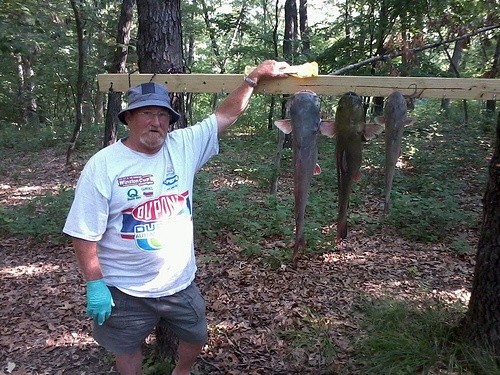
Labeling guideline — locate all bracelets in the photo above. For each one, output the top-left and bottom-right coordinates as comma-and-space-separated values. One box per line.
242, 75, 258, 87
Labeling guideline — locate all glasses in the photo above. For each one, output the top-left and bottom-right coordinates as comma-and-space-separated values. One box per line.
130, 109, 171, 117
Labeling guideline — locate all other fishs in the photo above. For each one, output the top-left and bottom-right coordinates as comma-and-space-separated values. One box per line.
274, 89, 321, 260
374, 91, 417, 214
321, 91, 385, 243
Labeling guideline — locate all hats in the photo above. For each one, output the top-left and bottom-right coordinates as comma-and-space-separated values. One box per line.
117, 82, 181, 125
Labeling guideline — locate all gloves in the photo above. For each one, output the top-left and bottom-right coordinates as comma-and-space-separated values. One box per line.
86, 278, 115, 327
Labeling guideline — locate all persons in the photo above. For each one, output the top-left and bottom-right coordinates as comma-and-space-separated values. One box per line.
62, 60, 289, 375
